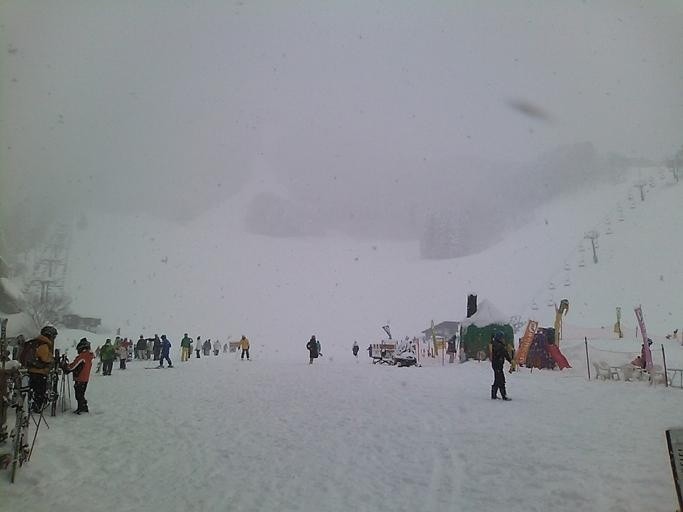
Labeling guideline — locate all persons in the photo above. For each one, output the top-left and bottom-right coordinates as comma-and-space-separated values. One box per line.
641, 344, 649, 368
95, 332, 250, 375
18, 326, 59, 414
316, 341, 322, 356
352, 341, 359, 355
367, 344, 372, 357
61, 338, 94, 414
491, 331, 513, 401
306, 336, 318, 365
447, 335, 457, 363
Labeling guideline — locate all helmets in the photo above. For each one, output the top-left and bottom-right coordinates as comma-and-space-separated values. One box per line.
41, 326, 57, 338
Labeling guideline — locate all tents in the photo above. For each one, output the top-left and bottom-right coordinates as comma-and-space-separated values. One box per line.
460, 299, 514, 360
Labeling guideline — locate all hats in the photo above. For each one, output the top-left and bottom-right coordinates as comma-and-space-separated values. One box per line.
76, 338, 90, 351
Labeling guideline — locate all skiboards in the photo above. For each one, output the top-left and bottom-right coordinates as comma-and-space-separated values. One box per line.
1, 317, 34, 485
51, 348, 72, 417
145, 365, 174, 369
